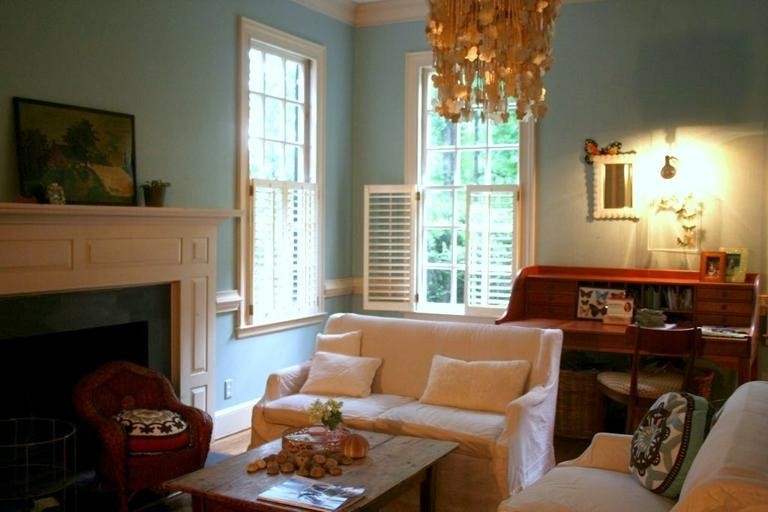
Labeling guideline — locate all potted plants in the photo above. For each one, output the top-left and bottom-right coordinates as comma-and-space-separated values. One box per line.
138, 178, 172, 205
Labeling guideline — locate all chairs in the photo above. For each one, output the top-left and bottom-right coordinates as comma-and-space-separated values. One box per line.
593, 322, 706, 435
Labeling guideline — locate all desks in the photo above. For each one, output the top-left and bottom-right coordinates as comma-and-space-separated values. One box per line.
161, 420, 465, 512
495, 265, 761, 398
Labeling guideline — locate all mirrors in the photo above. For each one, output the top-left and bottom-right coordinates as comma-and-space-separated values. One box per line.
593, 150, 644, 223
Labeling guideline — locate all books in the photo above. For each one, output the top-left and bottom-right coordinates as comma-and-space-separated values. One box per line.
256, 475, 367, 512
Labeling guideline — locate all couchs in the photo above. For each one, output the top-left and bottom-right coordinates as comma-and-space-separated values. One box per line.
78, 359, 214, 512
245, 308, 564, 512
494, 369, 767, 511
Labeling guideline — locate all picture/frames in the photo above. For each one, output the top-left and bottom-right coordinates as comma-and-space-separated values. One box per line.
719, 244, 750, 286
6, 89, 138, 210
698, 250, 728, 284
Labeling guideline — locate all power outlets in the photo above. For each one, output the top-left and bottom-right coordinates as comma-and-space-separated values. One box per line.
222, 378, 234, 399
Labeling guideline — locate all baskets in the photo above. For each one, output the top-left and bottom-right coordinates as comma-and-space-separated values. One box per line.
555, 369, 607, 440
631, 369, 714, 434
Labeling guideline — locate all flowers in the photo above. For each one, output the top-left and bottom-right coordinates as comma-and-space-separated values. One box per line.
306, 397, 344, 424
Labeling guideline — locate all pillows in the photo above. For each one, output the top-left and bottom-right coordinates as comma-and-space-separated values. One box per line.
315, 327, 366, 355
416, 354, 529, 416
108, 406, 189, 451
297, 351, 382, 403
626, 389, 712, 503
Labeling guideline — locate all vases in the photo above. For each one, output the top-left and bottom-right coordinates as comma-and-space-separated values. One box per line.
318, 425, 349, 452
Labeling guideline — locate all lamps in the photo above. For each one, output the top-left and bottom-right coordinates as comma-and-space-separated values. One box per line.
659, 152, 681, 183
422, 0, 565, 127
